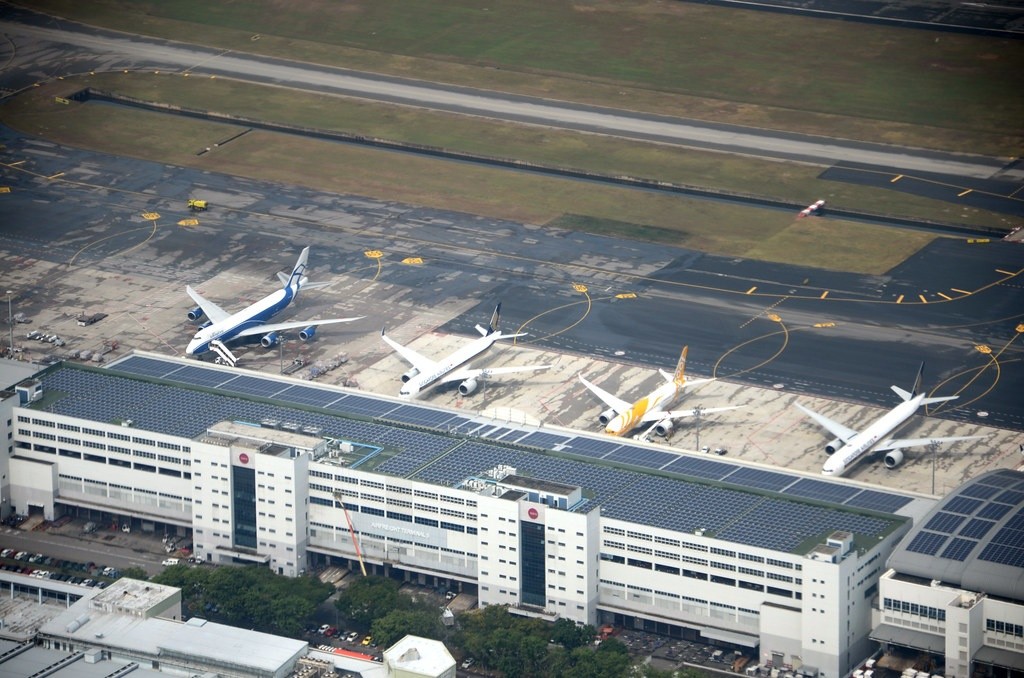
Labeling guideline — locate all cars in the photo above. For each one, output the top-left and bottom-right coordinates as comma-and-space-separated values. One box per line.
0, 548, 118, 588
122, 524, 131, 533
42, 349, 104, 373
304, 622, 377, 647
605, 624, 738, 666
162, 534, 205, 565
26, 332, 60, 343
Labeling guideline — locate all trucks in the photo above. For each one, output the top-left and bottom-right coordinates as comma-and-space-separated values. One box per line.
188, 199, 209, 211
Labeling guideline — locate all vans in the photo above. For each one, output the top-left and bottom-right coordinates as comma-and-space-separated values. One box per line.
161, 558, 181, 566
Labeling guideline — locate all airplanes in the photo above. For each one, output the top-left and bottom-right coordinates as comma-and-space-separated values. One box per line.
578, 344, 749, 444
792, 360, 989, 480
178, 246, 367, 371
380, 302, 553, 401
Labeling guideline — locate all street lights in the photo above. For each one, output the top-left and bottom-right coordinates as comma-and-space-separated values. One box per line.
6, 290, 14, 358
